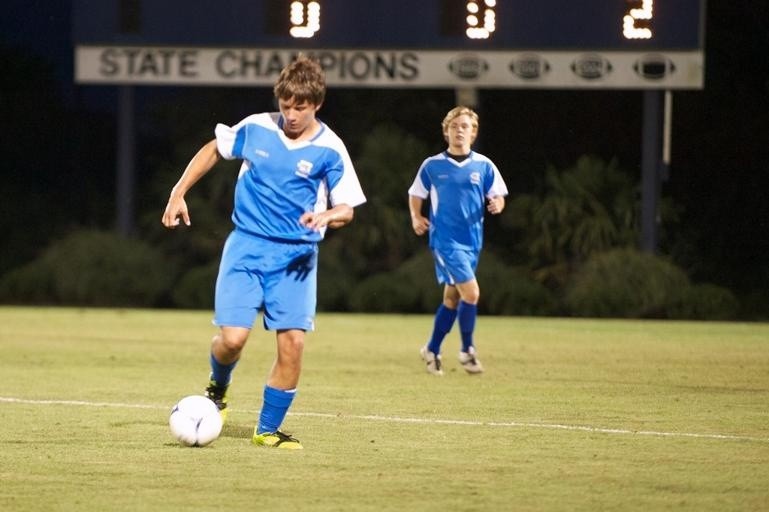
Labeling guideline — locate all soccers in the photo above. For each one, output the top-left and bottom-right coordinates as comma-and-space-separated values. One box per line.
167, 393, 223, 448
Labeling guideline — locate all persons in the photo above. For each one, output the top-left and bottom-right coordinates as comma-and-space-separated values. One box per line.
158, 56, 369, 453
406, 104, 510, 379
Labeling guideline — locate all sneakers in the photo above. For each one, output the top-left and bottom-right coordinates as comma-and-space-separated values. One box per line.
204, 373, 230, 432
252, 425, 304, 450
457, 346, 483, 373
420, 345, 444, 377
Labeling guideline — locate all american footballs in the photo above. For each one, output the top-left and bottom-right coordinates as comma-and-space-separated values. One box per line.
571, 53, 613, 79
510, 53, 551, 79
634, 54, 675, 80
448, 57, 488, 79
